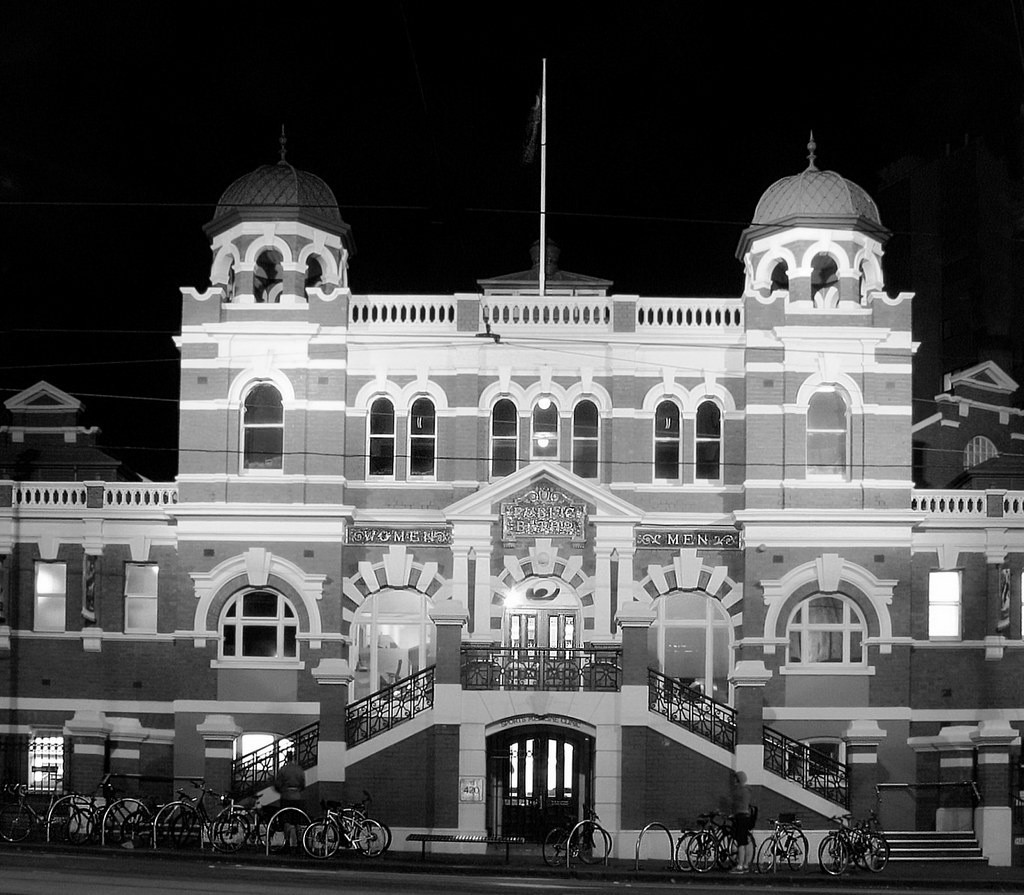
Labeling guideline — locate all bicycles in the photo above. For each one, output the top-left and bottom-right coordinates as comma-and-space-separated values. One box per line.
0, 771, 394, 858
540, 801, 613, 866
670, 803, 890, 876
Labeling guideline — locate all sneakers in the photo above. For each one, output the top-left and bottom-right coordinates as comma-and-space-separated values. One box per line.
729, 867, 743, 874
743, 867, 749, 872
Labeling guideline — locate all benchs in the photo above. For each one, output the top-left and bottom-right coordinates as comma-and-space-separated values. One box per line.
405, 832, 526, 865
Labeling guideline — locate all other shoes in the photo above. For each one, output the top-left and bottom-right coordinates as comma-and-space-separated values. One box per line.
276, 843, 292, 854
296, 845, 305, 855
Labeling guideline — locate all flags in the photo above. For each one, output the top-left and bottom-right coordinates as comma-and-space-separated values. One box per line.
520, 84, 542, 164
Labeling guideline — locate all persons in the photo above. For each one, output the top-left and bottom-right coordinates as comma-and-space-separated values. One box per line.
275, 752, 305, 854
729, 772, 751, 875
